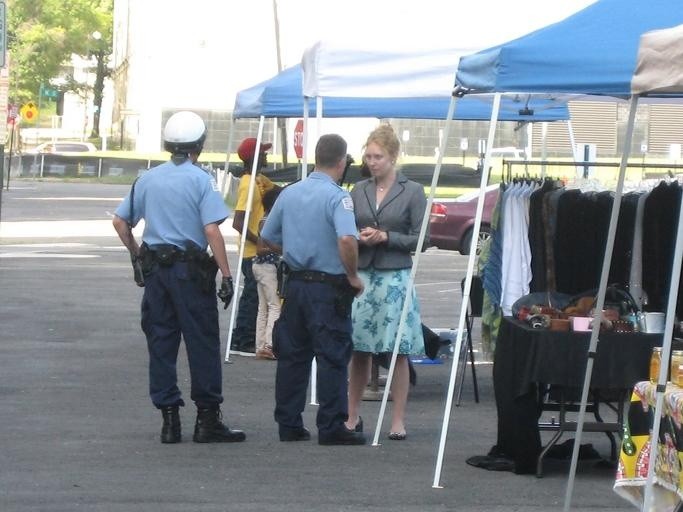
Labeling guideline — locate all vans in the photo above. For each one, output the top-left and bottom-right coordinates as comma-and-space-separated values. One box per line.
486, 147, 527, 159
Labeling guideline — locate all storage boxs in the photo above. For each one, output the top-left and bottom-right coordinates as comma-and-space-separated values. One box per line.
414, 322, 470, 359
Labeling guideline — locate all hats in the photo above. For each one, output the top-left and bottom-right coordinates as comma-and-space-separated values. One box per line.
238, 138, 272, 160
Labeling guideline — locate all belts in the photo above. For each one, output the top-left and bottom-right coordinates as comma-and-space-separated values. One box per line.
287, 269, 349, 287
140, 251, 209, 263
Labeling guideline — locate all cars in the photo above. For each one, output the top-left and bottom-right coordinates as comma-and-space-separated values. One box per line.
27, 142, 98, 155
423, 184, 504, 257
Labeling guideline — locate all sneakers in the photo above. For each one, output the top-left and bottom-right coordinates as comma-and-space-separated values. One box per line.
256, 346, 275, 360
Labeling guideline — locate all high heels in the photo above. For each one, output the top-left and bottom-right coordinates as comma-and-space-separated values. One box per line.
351, 415, 406, 440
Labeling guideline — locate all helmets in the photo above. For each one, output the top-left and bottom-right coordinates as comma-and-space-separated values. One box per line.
164, 111, 208, 153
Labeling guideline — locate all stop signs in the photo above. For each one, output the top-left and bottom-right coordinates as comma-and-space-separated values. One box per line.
293, 120, 303, 159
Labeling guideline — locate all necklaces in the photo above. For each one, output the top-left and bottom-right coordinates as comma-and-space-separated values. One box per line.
377, 181, 386, 192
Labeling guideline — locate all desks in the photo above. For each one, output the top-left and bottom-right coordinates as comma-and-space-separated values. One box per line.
463, 314, 683, 479
633, 379, 683, 512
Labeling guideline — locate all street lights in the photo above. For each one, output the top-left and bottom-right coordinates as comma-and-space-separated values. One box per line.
83, 31, 102, 139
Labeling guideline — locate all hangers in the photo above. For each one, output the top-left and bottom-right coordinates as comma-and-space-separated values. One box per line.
501, 161, 565, 194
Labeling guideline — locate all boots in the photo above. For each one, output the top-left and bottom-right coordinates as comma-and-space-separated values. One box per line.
161, 405, 245, 443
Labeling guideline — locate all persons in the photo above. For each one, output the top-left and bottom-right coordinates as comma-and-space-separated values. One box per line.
228, 137, 281, 359
260, 133, 368, 445
346, 126, 427, 439
251, 187, 282, 359
110, 111, 246, 446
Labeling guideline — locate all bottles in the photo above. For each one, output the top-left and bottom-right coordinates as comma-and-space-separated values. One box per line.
649, 346, 683, 388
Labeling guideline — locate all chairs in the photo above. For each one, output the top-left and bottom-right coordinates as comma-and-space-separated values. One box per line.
510, 290, 618, 465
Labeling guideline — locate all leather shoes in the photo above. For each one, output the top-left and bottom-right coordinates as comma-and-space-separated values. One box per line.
281, 428, 367, 445
